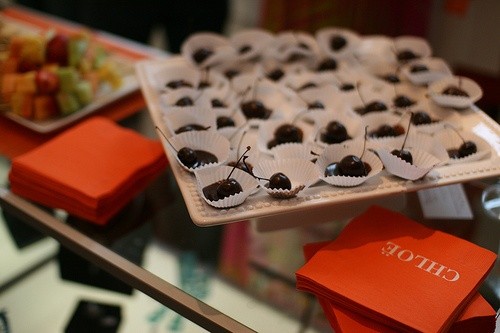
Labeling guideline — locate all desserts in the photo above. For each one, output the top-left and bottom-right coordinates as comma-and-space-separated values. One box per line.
150, 27, 483, 208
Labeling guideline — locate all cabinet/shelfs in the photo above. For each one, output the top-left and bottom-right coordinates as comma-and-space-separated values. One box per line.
0, 0, 499, 333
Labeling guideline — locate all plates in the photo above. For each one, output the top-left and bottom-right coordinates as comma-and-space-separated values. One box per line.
1, 26, 140, 133
135, 44, 500, 228
